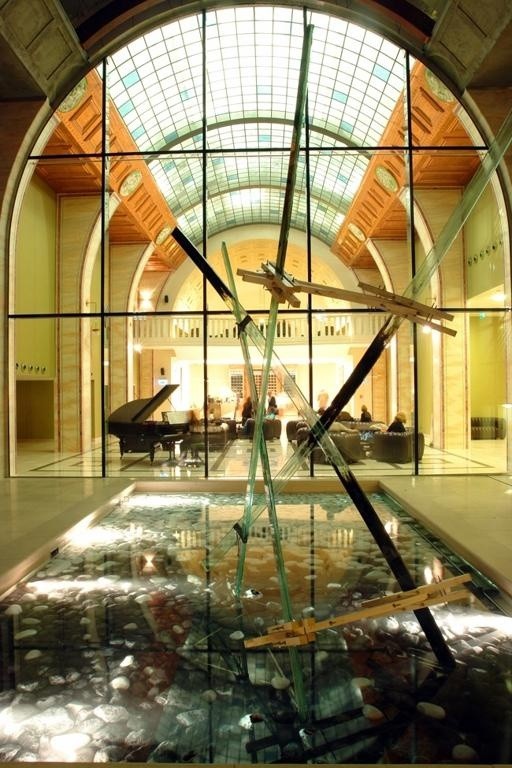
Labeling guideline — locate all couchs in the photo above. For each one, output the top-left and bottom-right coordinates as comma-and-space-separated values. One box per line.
185, 418, 425, 464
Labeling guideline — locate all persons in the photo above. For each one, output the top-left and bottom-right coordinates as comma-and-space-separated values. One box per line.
268, 392, 275, 407
387, 417, 406, 432
360, 406, 371, 422
241, 397, 252, 426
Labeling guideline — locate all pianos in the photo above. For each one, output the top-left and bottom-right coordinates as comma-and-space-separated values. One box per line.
107, 384, 189, 464
